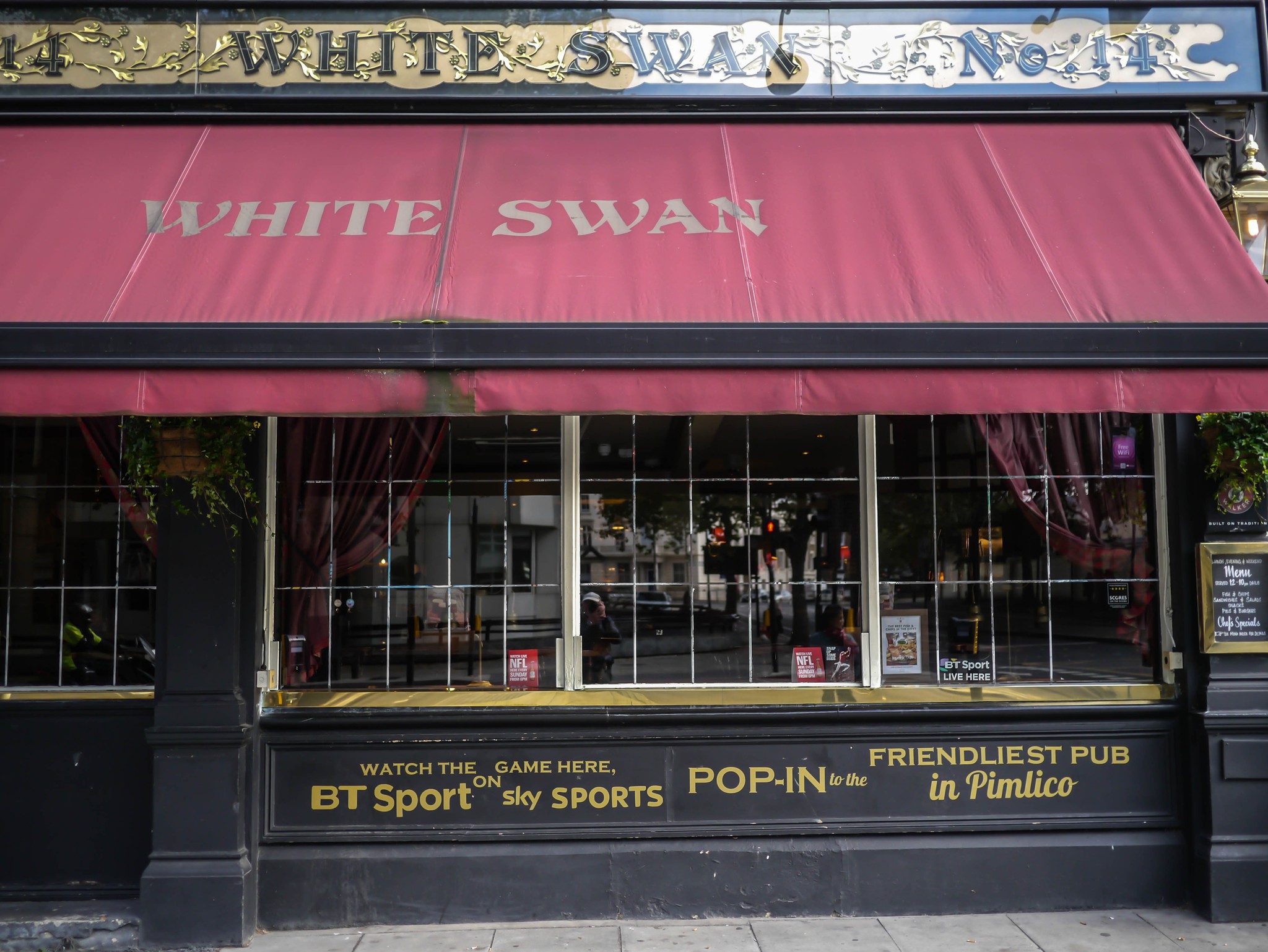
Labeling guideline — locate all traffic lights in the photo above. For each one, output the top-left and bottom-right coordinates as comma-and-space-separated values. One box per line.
762, 520, 780, 561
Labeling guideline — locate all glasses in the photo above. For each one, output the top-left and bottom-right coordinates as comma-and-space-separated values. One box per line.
836, 616, 842, 620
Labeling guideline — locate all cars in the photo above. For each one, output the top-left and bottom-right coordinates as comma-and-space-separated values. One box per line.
636, 590, 673, 613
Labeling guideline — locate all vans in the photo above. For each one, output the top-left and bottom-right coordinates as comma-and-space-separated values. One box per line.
581, 581, 636, 611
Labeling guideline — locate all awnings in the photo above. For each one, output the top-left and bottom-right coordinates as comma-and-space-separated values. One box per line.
6, 118, 1267, 428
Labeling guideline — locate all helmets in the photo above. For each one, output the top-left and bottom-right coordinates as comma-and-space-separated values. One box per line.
72, 604, 93, 626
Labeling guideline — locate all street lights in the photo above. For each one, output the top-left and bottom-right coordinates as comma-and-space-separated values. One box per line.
380, 559, 387, 621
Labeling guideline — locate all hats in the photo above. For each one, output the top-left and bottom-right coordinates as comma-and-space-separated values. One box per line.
582, 592, 601, 602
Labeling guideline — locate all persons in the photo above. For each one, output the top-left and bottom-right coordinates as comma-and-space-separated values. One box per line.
581, 595, 623, 682
810, 603, 859, 668
